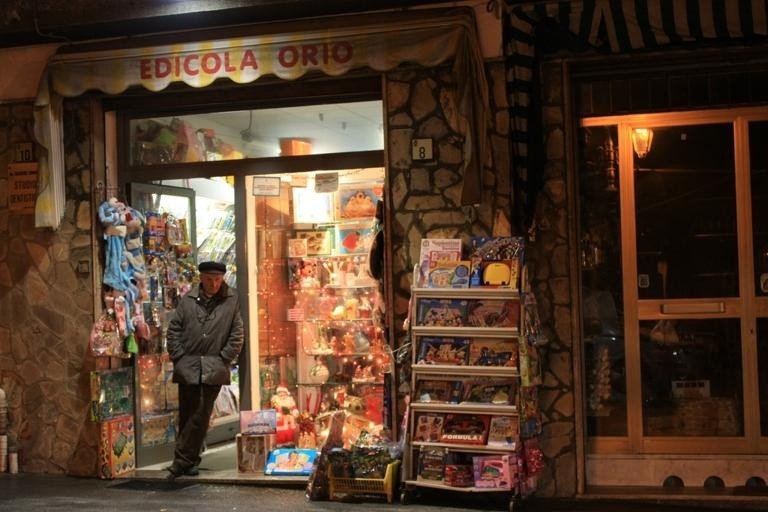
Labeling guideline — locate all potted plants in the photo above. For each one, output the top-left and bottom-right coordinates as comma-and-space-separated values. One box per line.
293, 228, 332, 255
333, 183, 383, 218
338, 225, 371, 252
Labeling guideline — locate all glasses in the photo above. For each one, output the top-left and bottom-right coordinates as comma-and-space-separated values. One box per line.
632, 128, 653, 157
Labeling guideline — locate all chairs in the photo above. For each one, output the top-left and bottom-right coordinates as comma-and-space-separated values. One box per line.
186, 468, 204, 478
166, 464, 184, 479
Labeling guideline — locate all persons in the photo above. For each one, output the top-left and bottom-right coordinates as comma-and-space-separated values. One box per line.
166, 260, 245, 476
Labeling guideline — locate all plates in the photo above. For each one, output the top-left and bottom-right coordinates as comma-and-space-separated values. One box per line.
400, 262, 536, 511
286, 221, 393, 449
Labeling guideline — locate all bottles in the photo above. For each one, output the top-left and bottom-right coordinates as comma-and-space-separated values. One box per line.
198, 260, 229, 275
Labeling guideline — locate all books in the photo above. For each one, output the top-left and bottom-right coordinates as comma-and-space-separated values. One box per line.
280, 138, 312, 156
